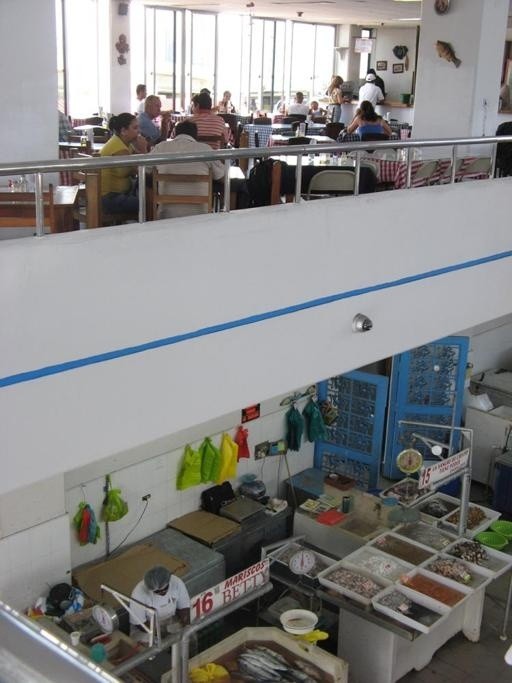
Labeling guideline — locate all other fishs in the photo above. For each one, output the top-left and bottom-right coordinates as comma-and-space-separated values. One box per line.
237, 645, 290, 681
288, 667, 316, 683
436, 40, 462, 68
294, 659, 323, 680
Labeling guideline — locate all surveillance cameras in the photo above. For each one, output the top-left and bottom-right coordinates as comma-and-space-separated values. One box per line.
352, 313, 373, 333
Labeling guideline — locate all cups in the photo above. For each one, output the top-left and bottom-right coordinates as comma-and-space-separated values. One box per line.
70, 631, 81, 646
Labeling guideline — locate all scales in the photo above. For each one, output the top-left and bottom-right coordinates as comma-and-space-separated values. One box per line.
262, 534, 317, 611
396, 432, 453, 502
91, 585, 161, 660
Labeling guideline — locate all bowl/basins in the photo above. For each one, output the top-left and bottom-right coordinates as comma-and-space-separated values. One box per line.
473, 531, 508, 551
490, 519, 512, 541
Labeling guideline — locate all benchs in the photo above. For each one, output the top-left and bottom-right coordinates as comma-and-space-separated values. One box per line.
0, 184, 81, 233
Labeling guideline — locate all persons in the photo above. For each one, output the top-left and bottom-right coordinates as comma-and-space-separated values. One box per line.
135, 85, 147, 113
98, 114, 147, 218
136, 94, 171, 145
219, 91, 235, 113
131, 567, 190, 644
139, 122, 229, 219
309, 100, 327, 119
286, 92, 309, 115
170, 93, 232, 152
359, 74, 385, 111
191, 88, 211, 115
325, 75, 344, 123
344, 101, 392, 142
366, 68, 385, 94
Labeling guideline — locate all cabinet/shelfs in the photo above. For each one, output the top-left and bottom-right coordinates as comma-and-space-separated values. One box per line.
316, 585, 486, 683
74, 527, 226, 616
176, 495, 291, 572
317, 99, 413, 135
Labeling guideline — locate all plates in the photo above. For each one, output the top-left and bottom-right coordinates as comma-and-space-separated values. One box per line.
440, 502, 502, 539
266, 541, 339, 579
409, 491, 461, 525
394, 566, 474, 614
317, 559, 394, 605
342, 544, 416, 584
364, 529, 438, 566
370, 583, 452, 634
417, 551, 494, 594
440, 537, 512, 579
390, 519, 461, 553
379, 476, 436, 508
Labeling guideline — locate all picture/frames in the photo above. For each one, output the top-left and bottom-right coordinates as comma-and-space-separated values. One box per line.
393, 63, 403, 73
376, 60, 387, 71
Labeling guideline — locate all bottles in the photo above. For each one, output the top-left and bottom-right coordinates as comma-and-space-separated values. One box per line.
7, 174, 36, 193
80, 131, 88, 147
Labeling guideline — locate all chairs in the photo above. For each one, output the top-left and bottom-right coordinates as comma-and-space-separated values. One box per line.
219, 114, 239, 147
239, 131, 250, 174
362, 132, 389, 140
307, 169, 355, 200
459, 156, 491, 181
441, 158, 464, 182
414, 160, 439, 187
194, 135, 226, 166
326, 122, 344, 138
152, 165, 215, 218
284, 114, 306, 123
67, 148, 140, 230
254, 118, 271, 125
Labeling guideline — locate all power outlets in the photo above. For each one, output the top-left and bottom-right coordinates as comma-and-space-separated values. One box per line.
255, 440, 269, 461
143, 494, 151, 502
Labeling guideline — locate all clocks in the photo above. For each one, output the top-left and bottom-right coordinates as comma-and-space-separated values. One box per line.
397, 448, 423, 474
434, 0, 450, 14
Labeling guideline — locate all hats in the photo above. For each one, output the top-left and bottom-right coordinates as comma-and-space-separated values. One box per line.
366, 73, 376, 81
144, 566, 171, 591
